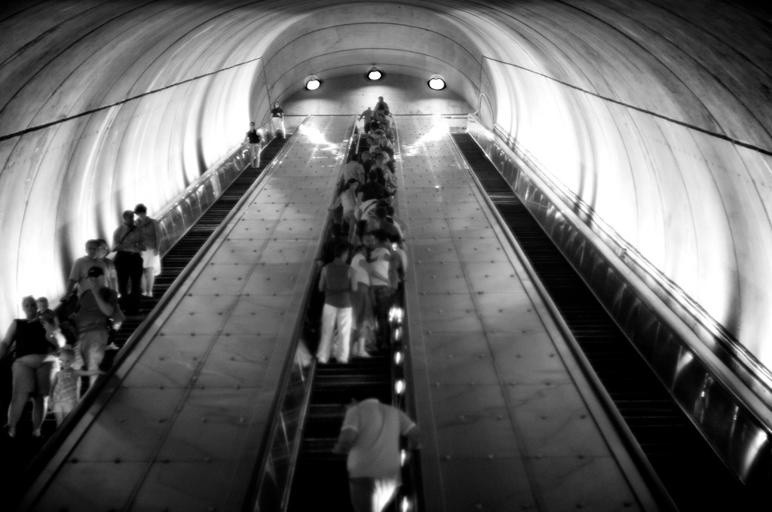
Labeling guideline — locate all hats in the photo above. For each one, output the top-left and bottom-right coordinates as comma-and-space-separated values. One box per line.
84, 265, 105, 280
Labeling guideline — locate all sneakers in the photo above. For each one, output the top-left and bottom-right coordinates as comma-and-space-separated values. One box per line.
104, 342, 119, 351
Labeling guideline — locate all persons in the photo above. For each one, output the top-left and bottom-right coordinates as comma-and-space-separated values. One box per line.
270, 101, 286, 139
241, 120, 262, 170
0, 202, 164, 437
312, 95, 408, 367
328, 385, 423, 510
